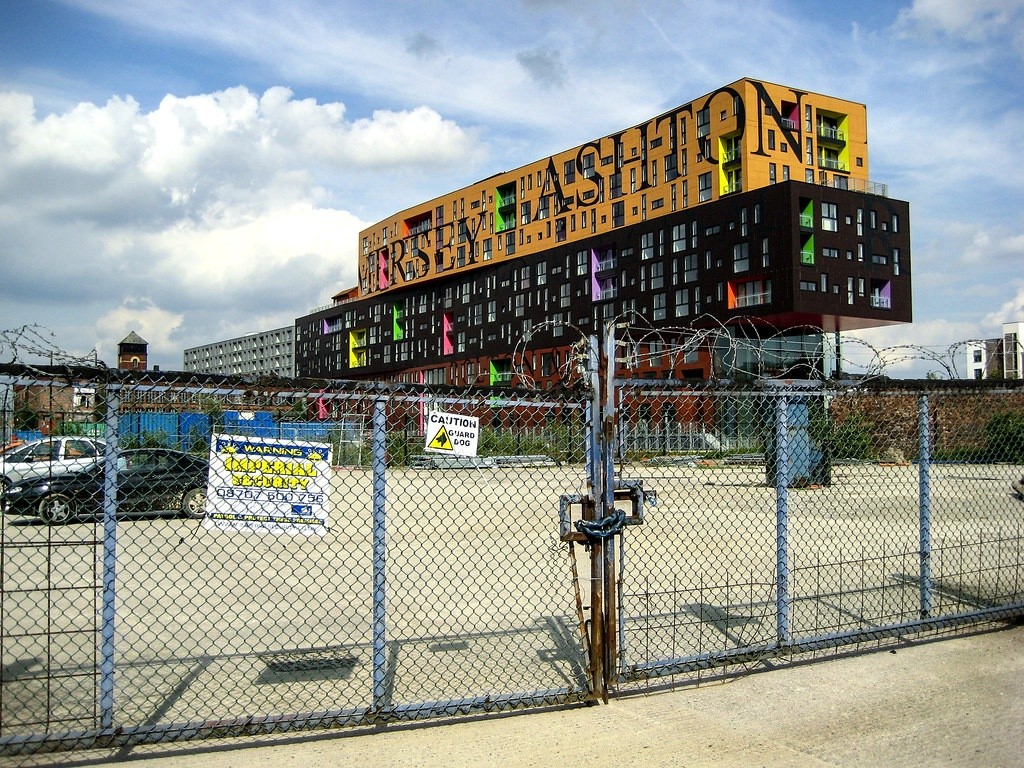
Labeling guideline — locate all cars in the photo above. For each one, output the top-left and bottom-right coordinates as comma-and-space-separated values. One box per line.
0, 435, 133, 505
0, 448, 209, 523
0, 440, 83, 462
